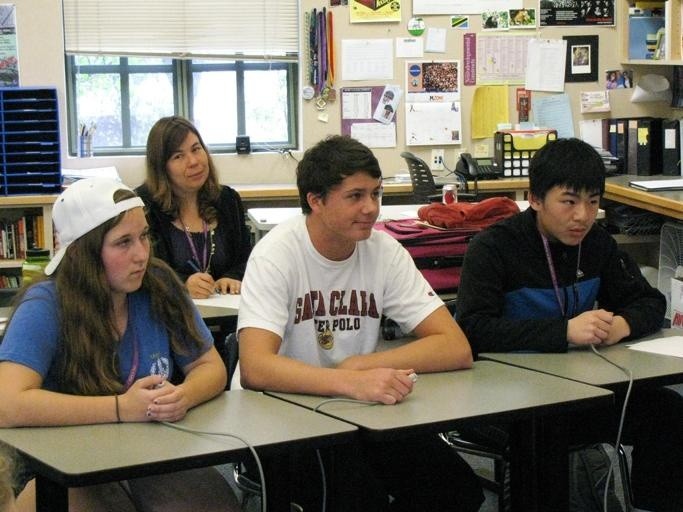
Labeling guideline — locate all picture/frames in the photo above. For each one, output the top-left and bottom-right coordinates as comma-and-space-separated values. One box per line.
562, 35, 598, 82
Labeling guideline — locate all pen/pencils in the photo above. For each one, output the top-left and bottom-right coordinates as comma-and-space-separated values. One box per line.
187, 260, 221, 294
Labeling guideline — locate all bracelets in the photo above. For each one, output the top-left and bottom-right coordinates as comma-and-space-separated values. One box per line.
114, 392, 124, 423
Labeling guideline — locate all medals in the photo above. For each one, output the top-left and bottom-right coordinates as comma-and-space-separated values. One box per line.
301, 85, 336, 112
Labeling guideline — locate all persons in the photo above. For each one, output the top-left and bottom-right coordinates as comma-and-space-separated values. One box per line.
605, 71, 617, 89
453, 137, 683, 512
131, 114, 253, 392
621, 71, 633, 89
0, 167, 244, 512
228, 134, 491, 512
579, 1, 613, 18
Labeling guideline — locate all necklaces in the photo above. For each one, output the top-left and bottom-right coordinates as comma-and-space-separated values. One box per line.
185, 220, 216, 274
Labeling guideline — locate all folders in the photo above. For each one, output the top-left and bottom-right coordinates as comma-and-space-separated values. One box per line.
601, 118, 677, 176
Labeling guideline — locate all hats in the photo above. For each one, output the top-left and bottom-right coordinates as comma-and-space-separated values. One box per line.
42, 175, 148, 277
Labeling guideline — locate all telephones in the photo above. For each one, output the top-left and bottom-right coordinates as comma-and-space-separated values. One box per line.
457, 154, 503, 177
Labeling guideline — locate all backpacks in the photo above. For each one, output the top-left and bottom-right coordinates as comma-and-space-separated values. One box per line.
568, 443, 625, 512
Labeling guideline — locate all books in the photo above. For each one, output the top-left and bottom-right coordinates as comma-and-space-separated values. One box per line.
0, 211, 44, 291
627, 179, 683, 193
591, 117, 683, 176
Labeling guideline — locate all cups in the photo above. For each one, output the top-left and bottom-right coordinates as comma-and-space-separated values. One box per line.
646, 33, 658, 60
76, 134, 94, 159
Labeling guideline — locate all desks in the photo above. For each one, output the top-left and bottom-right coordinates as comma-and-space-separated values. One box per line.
0, 390, 359, 512
479, 329, 683, 394
264, 359, 613, 442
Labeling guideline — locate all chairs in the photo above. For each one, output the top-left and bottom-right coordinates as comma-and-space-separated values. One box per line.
400, 152, 477, 204
